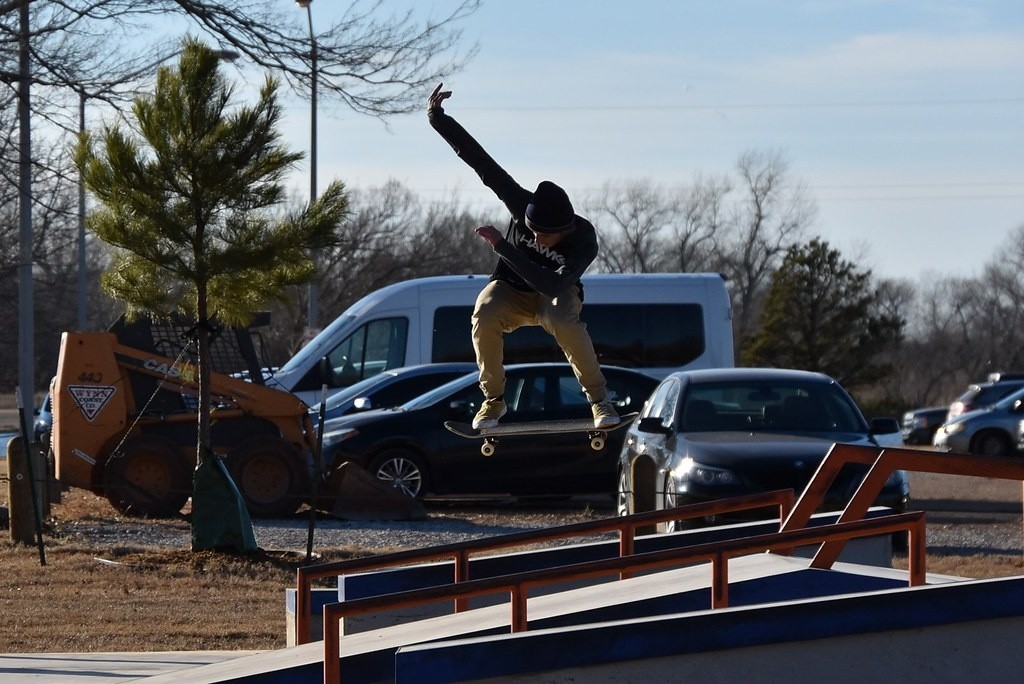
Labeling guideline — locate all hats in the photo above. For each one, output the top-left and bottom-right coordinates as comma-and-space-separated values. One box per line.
525, 181, 575, 233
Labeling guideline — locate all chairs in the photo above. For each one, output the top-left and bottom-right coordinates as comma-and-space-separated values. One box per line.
456, 388, 841, 432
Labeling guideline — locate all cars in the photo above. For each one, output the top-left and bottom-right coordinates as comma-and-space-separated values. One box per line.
932, 388, 1024, 458
220, 356, 389, 408
947, 379, 1024, 424
32, 391, 52, 433
902, 406, 952, 446
615, 368, 909, 553
307, 362, 487, 428
305, 362, 742, 526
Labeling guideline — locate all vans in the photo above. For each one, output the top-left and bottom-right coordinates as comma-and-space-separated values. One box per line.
264, 274, 738, 410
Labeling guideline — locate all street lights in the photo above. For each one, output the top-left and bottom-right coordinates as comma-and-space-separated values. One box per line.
294, 0, 320, 334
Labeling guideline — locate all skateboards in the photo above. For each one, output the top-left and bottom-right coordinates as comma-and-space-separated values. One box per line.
443, 410, 639, 456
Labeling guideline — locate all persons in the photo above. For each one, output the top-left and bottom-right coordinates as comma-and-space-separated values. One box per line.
428, 82, 622, 429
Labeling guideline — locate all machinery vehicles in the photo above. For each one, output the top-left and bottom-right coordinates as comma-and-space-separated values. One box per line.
41, 307, 320, 531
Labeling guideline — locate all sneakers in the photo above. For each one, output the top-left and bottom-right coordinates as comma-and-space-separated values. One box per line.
591, 402, 620, 428
473, 400, 507, 429
